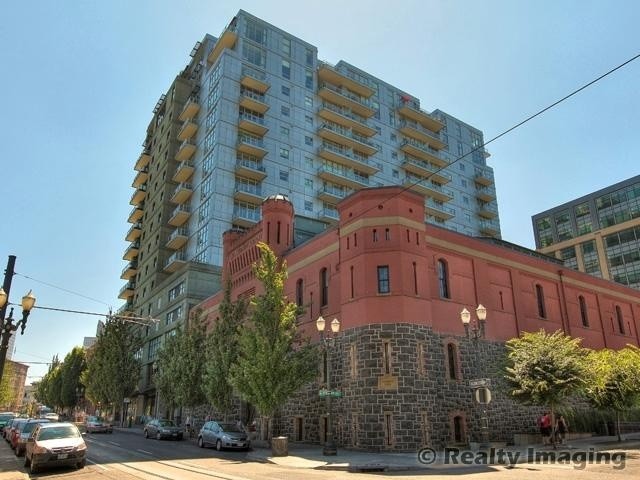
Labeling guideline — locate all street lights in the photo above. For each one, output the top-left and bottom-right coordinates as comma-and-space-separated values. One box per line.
0, 288, 36, 383
460, 304, 490, 451
316, 316, 341, 456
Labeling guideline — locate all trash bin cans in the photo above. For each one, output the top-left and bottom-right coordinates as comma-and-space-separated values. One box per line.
271, 436, 289, 456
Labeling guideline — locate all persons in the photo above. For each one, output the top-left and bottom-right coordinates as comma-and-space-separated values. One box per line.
539, 413, 552, 446
553, 412, 568, 446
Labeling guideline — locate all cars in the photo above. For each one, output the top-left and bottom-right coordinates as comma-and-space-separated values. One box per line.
84, 416, 113, 434
0, 407, 89, 474
143, 419, 183, 440
197, 421, 252, 451
